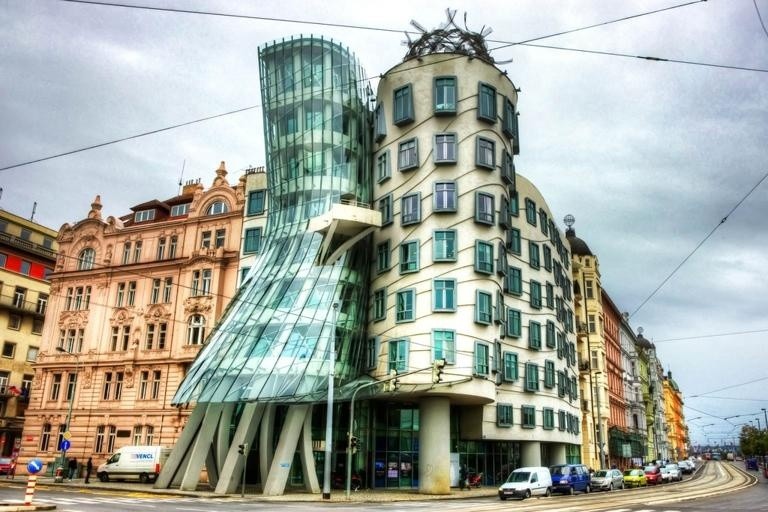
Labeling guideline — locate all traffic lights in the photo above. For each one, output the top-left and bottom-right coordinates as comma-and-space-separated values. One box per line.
351, 437, 356, 446
390, 369, 400, 392
432, 360, 445, 384
238, 444, 244, 454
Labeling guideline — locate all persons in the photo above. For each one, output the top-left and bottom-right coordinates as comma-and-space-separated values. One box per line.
6, 459, 16, 480
85, 457, 92, 483
460, 464, 468, 479
67, 457, 78, 482
611, 461, 617, 469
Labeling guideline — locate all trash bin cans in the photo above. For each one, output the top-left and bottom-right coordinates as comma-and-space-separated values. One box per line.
45, 456, 81, 478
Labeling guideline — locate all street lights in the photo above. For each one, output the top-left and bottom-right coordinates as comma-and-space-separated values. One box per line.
56, 347, 78, 483
749, 408, 768, 431
323, 303, 339, 497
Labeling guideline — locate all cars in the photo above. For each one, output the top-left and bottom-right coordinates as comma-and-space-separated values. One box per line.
744, 459, 758, 471
623, 469, 647, 487
0, 456, 13, 475
704, 453, 720, 460
678, 460, 695, 474
590, 469, 624, 491
639, 466, 662, 485
659, 468, 673, 482
666, 464, 682, 481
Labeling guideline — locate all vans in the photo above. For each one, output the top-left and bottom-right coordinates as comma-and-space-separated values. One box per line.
498, 467, 553, 500
549, 465, 591, 495
97, 445, 172, 482
